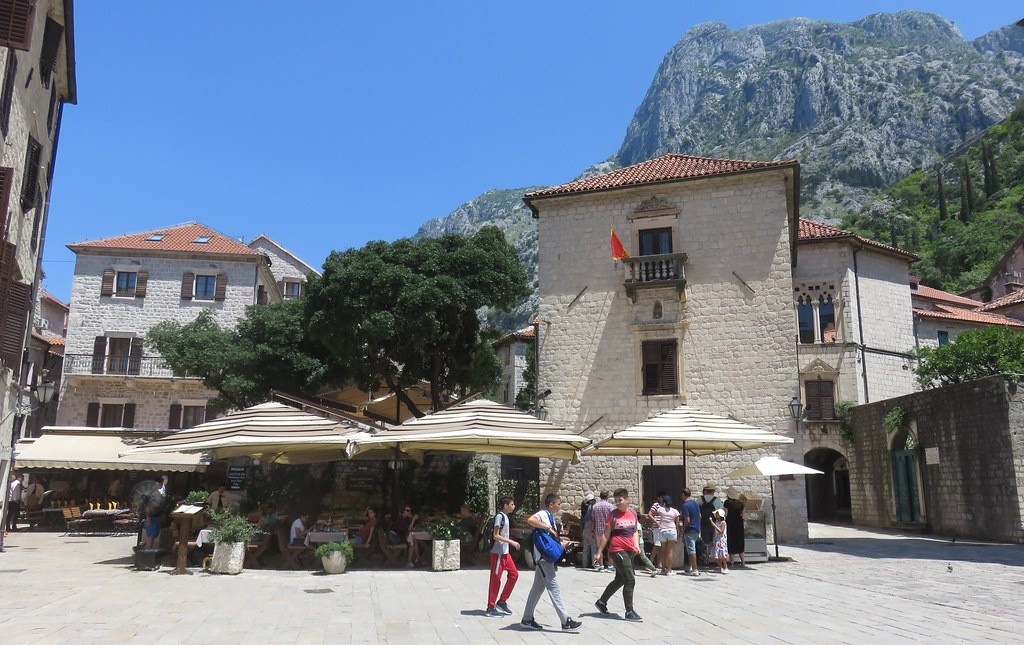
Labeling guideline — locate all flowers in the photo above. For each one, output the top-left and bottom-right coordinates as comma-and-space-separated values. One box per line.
427, 516, 467, 538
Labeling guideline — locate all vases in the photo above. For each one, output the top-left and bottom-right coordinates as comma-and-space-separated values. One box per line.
432, 538, 461, 570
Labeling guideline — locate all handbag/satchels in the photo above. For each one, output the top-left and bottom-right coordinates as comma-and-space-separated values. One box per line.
27, 494, 40, 505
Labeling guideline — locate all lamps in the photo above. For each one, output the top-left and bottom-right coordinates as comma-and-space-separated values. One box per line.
788, 395, 803, 433
20, 379, 57, 415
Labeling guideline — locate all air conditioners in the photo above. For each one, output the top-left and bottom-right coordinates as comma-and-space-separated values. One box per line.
35, 318, 48, 330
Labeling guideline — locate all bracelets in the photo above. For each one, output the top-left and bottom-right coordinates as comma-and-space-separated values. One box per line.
546, 526, 552, 530
305, 529, 310, 533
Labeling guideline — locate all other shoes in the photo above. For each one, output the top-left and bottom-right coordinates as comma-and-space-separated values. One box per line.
685, 569, 699, 576
406, 560, 415, 568
133, 542, 143, 552
651, 568, 662, 576
598, 565, 604, 573
667, 569, 676, 575
625, 610, 643, 622
607, 565, 616, 572
713, 567, 729, 574
645, 566, 652, 573
6, 527, 21, 532
658, 567, 667, 574
594, 599, 609, 615
415, 558, 427, 565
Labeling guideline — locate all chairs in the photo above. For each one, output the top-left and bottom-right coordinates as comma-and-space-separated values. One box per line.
49, 500, 132, 536
202, 517, 582, 570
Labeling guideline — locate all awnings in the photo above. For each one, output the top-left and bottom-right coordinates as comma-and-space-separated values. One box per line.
14, 426, 214, 473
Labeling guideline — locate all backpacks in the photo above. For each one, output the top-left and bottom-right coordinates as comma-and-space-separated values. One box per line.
482, 512, 505, 551
528, 509, 565, 578
700, 495, 718, 544
388, 529, 401, 544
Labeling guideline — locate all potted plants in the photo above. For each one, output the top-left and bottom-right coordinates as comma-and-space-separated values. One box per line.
128, 483, 165, 571
314, 540, 355, 574
198, 509, 264, 574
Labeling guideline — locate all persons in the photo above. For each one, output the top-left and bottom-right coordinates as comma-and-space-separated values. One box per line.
582, 484, 745, 577
521, 493, 582, 631
6, 474, 44, 531
485, 495, 521, 616
451, 505, 476, 557
156, 476, 169, 500
143, 506, 161, 549
205, 483, 232, 512
73, 475, 125, 502
594, 489, 644, 622
349, 503, 422, 568
250, 494, 316, 566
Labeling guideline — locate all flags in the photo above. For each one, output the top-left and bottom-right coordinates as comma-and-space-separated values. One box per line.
610, 229, 634, 267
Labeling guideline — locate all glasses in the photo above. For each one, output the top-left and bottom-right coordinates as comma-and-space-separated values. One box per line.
405, 509, 411, 512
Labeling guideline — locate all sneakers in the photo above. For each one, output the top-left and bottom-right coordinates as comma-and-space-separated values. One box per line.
496, 599, 512, 614
562, 617, 583, 632
520, 617, 543, 630
485, 607, 504, 618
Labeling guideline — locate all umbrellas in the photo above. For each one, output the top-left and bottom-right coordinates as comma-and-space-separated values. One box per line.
722, 457, 824, 562
581, 404, 795, 503
118, 401, 395, 464
352, 399, 593, 460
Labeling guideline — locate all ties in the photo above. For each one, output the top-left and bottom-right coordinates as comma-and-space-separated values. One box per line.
218, 494, 222, 507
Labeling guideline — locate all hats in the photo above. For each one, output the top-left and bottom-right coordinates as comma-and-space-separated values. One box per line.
586, 494, 598, 502
663, 495, 671, 502
725, 486, 739, 500
704, 480, 719, 490
712, 509, 726, 521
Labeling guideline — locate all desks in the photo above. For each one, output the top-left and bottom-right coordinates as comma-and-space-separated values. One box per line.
82, 508, 129, 536
304, 529, 350, 551
42, 505, 77, 530
